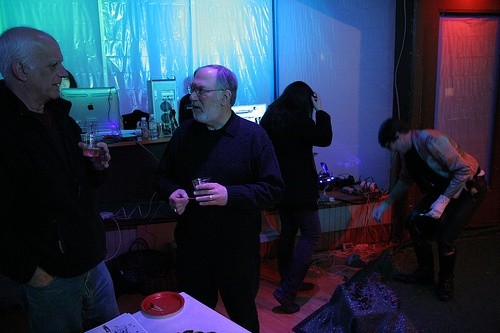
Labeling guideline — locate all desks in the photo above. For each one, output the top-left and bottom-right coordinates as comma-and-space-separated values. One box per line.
132, 292, 252, 333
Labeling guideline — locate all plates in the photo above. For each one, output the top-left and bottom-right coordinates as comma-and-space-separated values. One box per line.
141, 291, 185, 316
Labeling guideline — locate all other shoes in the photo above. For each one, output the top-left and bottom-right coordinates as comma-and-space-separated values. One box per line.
297, 282, 314, 291
433, 272, 454, 301
394, 265, 434, 285
273, 289, 300, 313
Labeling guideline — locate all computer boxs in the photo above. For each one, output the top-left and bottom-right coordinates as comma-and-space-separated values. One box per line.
148, 76, 177, 127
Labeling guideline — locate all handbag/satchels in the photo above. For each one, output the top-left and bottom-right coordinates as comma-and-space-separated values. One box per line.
111, 238, 167, 294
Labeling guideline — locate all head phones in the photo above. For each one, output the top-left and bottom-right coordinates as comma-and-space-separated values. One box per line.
360, 176, 376, 192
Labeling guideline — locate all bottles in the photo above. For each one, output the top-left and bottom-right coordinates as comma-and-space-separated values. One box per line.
141, 117, 149, 141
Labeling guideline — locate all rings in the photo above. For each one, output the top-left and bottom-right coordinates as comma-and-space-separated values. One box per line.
174, 208, 176, 213
210, 195, 213, 200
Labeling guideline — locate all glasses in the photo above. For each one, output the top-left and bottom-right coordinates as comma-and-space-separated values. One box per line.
187, 86, 226, 95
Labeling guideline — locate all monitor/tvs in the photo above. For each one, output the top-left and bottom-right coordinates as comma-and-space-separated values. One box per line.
60, 88, 120, 139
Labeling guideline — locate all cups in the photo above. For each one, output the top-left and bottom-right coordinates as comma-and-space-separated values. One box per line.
192, 177, 211, 190
149, 122, 158, 141
82, 117, 100, 158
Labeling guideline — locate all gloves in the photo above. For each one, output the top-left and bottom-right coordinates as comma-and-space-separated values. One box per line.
425, 195, 449, 219
373, 202, 388, 222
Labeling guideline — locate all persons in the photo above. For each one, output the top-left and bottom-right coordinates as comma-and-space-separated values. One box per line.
260, 81, 332, 312
160, 65, 284, 333
372, 118, 488, 302
0, 26, 119, 333
